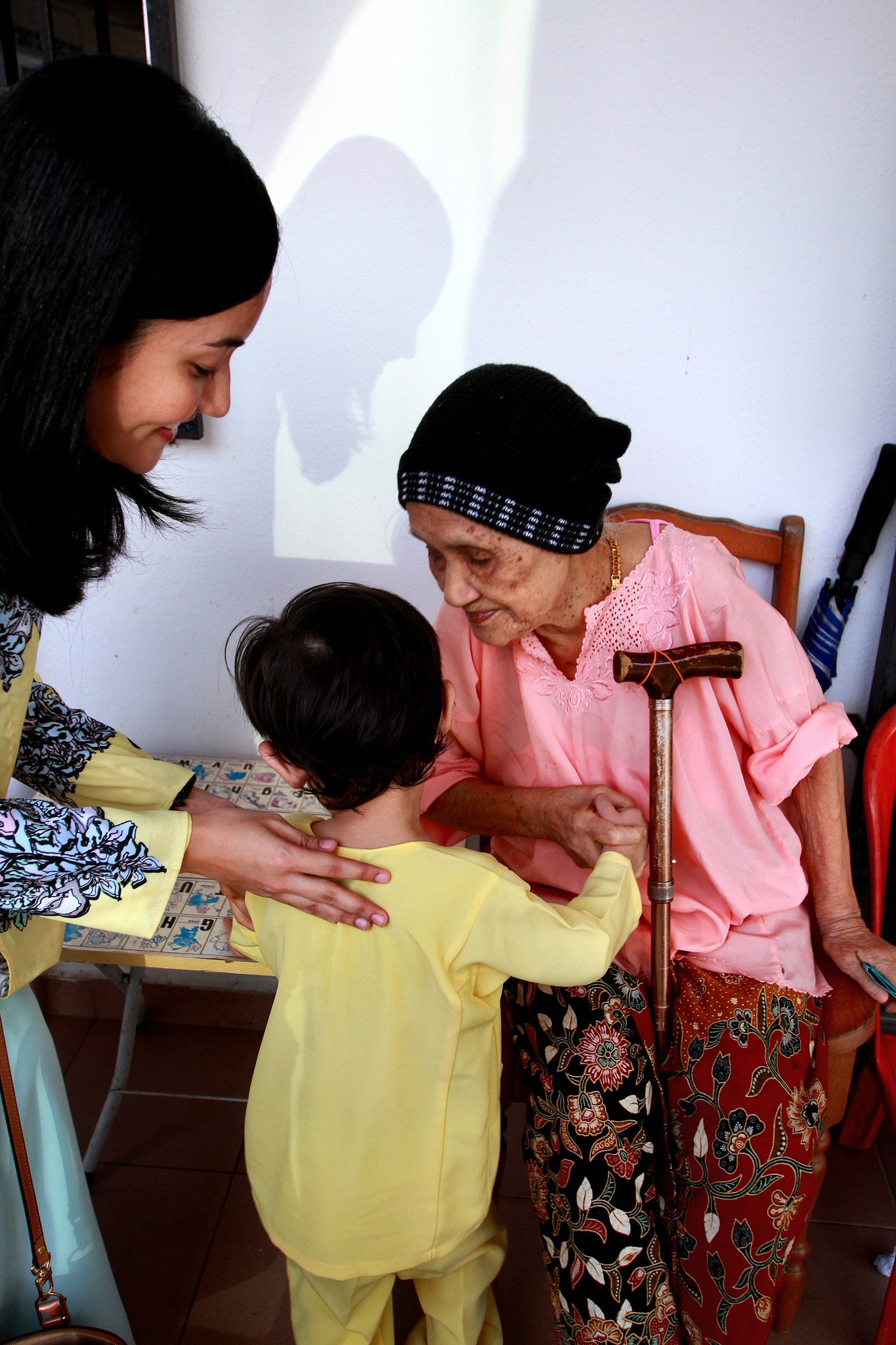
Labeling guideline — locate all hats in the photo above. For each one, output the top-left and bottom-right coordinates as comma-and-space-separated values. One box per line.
396, 364, 631, 555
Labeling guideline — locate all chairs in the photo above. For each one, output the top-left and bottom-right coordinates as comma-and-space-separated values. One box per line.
835, 702, 896, 1153
601, 497, 810, 641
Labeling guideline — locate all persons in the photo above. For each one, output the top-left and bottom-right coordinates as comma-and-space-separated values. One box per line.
222, 581, 650, 1345
396, 363, 896, 1345
0, 53, 394, 1345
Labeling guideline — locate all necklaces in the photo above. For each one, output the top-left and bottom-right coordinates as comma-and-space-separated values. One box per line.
597, 527, 621, 594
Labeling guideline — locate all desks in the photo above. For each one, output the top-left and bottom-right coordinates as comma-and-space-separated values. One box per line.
57, 754, 337, 1188
